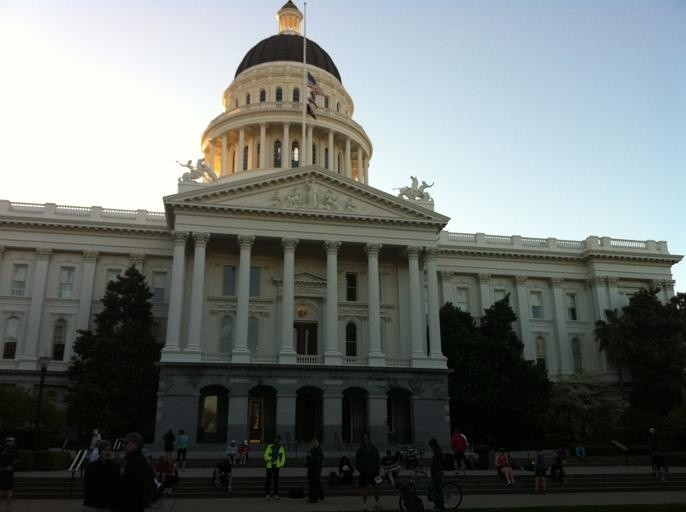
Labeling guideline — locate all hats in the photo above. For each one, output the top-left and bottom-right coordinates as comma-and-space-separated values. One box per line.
117, 432, 144, 448
94, 439, 113, 455
243, 439, 248, 446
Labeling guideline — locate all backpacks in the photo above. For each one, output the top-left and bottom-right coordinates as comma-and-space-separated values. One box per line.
328, 472, 338, 486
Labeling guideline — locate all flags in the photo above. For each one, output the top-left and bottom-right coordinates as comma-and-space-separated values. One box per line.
305, 70, 329, 101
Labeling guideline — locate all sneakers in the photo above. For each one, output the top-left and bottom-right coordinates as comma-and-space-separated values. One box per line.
265, 494, 280, 501
362, 503, 383, 511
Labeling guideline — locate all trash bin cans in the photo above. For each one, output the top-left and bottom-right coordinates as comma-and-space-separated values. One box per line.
476, 445, 490, 470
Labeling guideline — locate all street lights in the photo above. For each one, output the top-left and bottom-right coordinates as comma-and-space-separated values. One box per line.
648, 427, 656, 456
32, 345, 54, 449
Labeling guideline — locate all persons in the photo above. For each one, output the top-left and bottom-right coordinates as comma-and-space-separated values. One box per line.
204, 426, 211, 443
575, 442, 586, 464
213, 435, 286, 501
0, 435, 19, 509
428, 437, 446, 511
83, 425, 190, 511
495, 445, 516, 486
551, 456, 568, 485
451, 426, 471, 476
531, 445, 549, 495
306, 435, 402, 511
558, 446, 568, 464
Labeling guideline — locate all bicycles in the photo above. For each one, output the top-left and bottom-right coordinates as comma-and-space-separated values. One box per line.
396, 468, 466, 511
